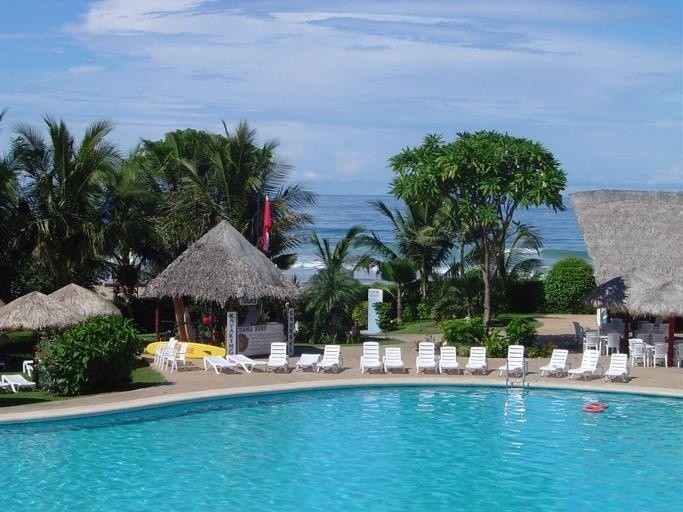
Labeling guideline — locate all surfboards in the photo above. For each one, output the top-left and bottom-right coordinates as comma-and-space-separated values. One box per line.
147, 342, 226, 358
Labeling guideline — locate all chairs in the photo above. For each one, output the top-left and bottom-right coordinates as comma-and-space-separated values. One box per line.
267, 342, 289, 373
466, 347, 489, 374
226, 354, 268, 374
296, 353, 321, 372
539, 349, 569, 377
569, 316, 683, 383
315, 344, 343, 374
153, 334, 188, 374
437, 346, 460, 374
498, 344, 528, 375
416, 341, 436, 375
383, 347, 405, 374
360, 341, 383, 374
204, 355, 236, 377
0, 371, 38, 395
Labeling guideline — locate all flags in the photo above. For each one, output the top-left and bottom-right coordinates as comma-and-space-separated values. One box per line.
261, 200, 271, 253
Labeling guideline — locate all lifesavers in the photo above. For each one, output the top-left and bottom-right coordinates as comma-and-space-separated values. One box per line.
583, 403, 608, 412
